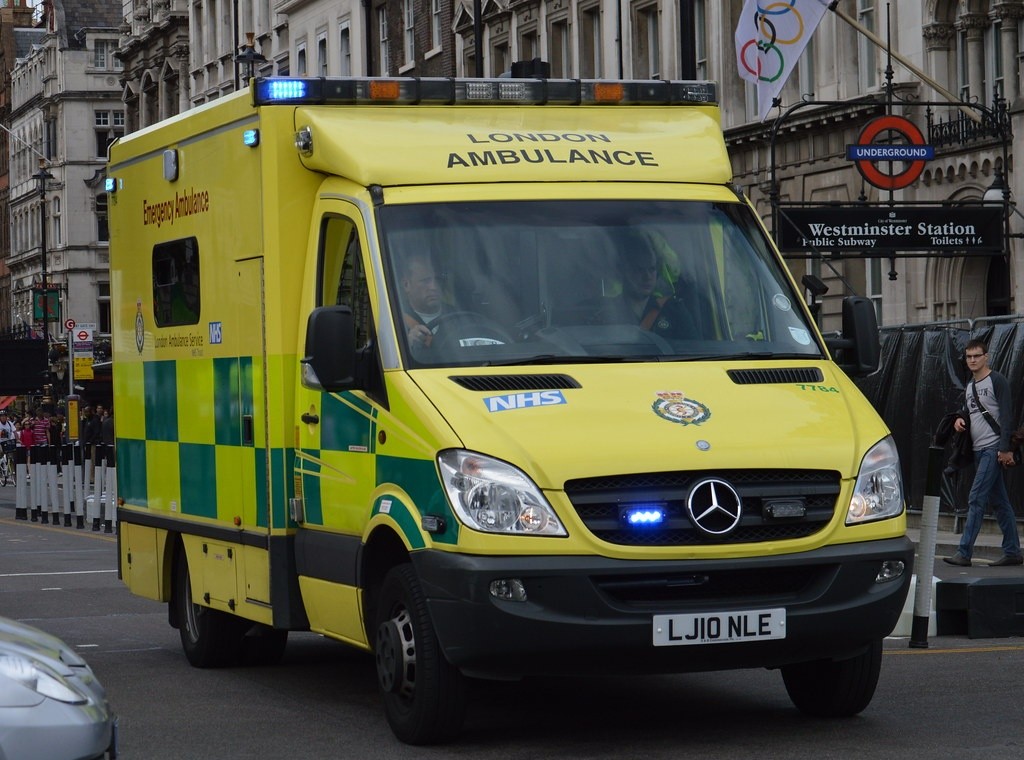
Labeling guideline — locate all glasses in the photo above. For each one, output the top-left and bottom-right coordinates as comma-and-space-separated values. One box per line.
966, 352, 986, 359
25, 423, 30, 425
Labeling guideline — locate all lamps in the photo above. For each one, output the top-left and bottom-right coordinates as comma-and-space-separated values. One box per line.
982, 166, 1015, 220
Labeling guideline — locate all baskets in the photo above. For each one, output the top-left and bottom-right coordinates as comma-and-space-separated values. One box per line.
0, 439, 17, 453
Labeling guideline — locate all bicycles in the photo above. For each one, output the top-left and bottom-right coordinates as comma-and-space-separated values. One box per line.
0, 437, 20, 487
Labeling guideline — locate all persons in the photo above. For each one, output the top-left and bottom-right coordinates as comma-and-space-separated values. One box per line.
393, 253, 463, 352
0, 403, 114, 468
941, 340, 1024, 566
598, 235, 670, 330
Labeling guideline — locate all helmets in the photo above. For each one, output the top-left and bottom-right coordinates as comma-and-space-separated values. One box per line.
0, 410, 7, 416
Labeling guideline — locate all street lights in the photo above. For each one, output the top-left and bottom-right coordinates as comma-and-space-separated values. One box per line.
31, 157, 55, 415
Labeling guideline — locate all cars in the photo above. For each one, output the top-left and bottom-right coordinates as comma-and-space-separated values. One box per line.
0, 615, 118, 760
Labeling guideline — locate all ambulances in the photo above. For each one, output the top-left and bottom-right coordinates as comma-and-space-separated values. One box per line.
104, 58, 913, 746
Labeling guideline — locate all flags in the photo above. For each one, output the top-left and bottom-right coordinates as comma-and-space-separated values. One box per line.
734, 0, 830, 124
22, 321, 41, 340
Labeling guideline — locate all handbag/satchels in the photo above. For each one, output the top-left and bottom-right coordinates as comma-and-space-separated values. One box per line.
1010, 431, 1024, 464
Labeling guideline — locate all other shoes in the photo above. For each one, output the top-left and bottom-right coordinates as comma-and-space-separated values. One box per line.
988, 554, 1024, 566
58, 473, 63, 478
943, 552, 972, 567
